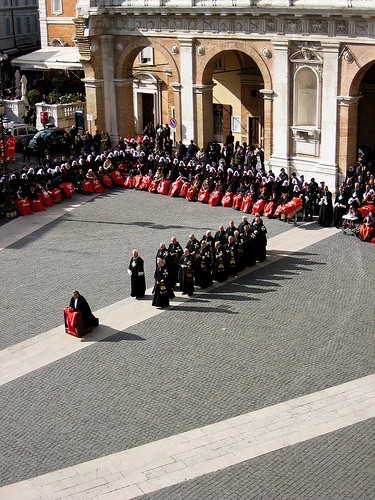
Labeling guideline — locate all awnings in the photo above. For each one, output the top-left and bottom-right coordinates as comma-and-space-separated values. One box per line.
12, 45, 85, 71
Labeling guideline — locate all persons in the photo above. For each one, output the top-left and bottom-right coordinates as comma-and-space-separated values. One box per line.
0, 105, 375, 307
67, 291, 93, 329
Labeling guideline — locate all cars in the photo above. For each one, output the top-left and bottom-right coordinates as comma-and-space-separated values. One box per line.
27, 127, 73, 158
11, 124, 39, 143
